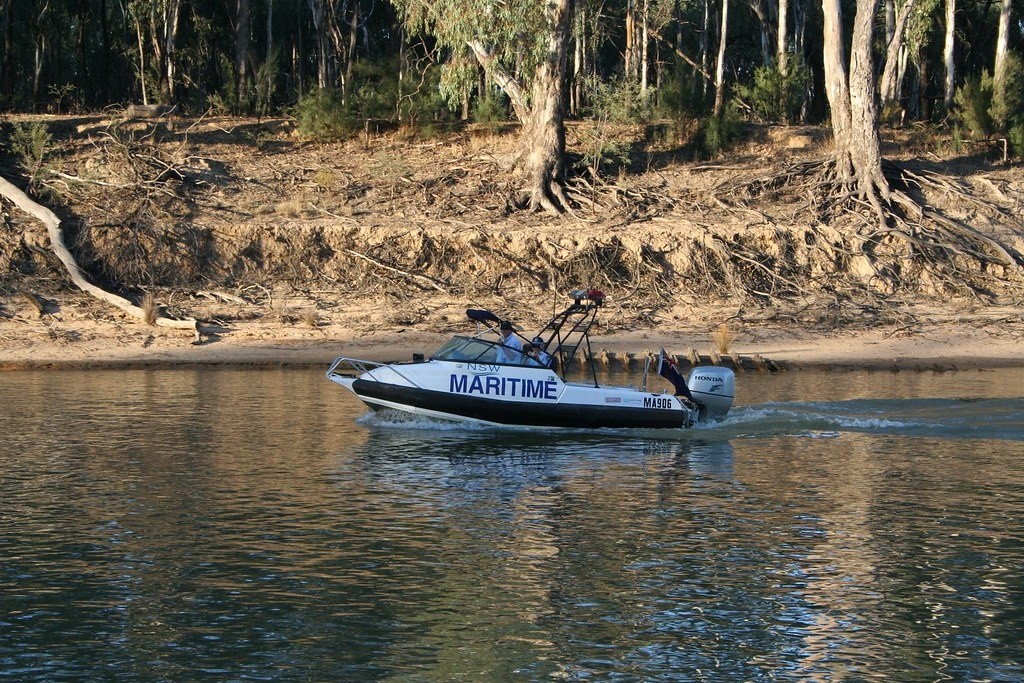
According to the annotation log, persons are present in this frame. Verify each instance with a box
[525,337,552,369]
[495,321,521,364]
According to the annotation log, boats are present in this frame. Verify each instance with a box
[325,289,734,429]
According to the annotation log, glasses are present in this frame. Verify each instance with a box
[500,327,508,330]
[532,345,540,348]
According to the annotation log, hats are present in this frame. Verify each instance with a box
[531,336,543,344]
[501,320,511,327]
